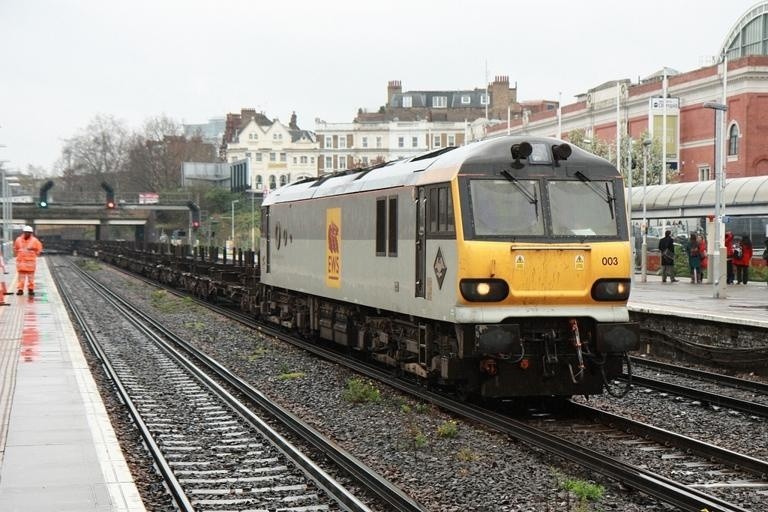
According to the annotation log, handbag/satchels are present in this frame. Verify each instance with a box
[664,248,677,261]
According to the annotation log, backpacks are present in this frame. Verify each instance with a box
[689,247,698,257]
[734,242,744,260]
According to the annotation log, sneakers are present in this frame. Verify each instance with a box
[15,288,36,296]
[689,277,703,284]
[727,279,748,285]
[670,279,680,281]
[662,279,666,282]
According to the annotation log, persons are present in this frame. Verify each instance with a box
[732,233,753,285]
[13,224,43,297]
[0,242,7,305]
[696,234,708,282]
[686,233,701,285]
[725,231,735,285]
[659,230,680,282]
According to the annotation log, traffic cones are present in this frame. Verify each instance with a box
[0,279,14,306]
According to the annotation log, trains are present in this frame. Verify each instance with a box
[42,134,640,404]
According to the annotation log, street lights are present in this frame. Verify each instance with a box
[641,139,653,282]
[703,102,728,299]
[251,167,263,253]
[232,199,240,241]
[0,144,22,263]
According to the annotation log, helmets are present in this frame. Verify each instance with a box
[22,225,34,233]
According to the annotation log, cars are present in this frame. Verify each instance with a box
[646,225,690,251]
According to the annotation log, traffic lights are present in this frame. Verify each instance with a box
[186,199,200,230]
[39,181,54,208]
[100,180,115,209]
